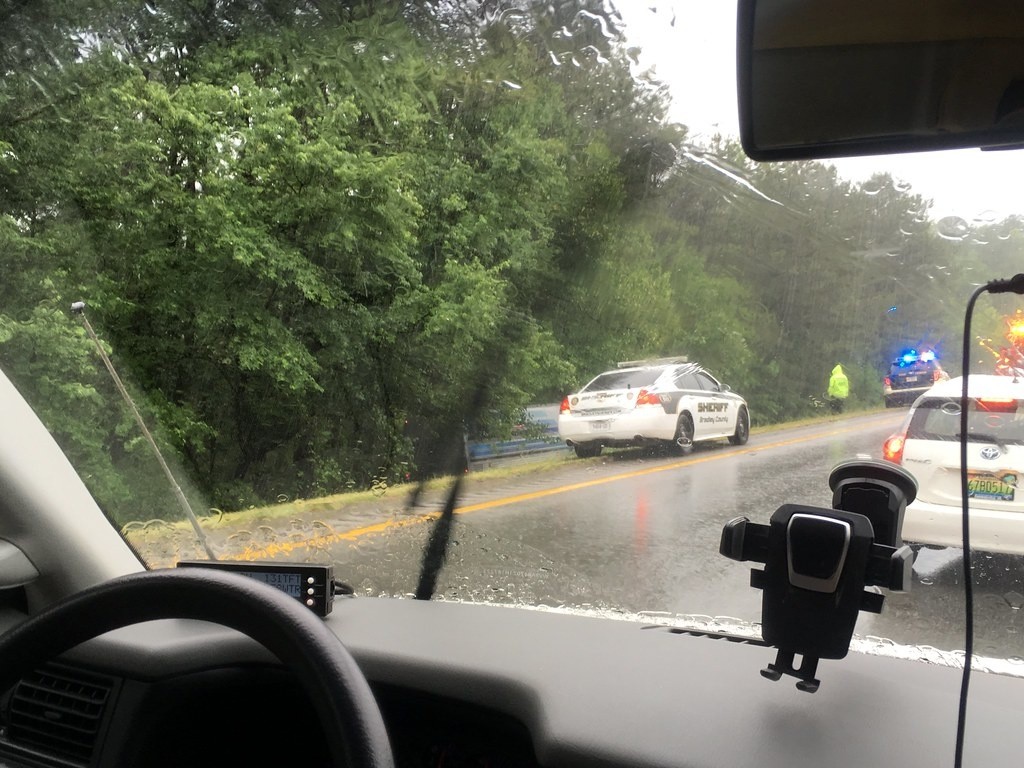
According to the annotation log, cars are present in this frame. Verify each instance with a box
[557,355,753,451]
[881,373,1024,563]
[883,354,949,406]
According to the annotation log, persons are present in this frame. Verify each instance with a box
[827,364,848,416]
